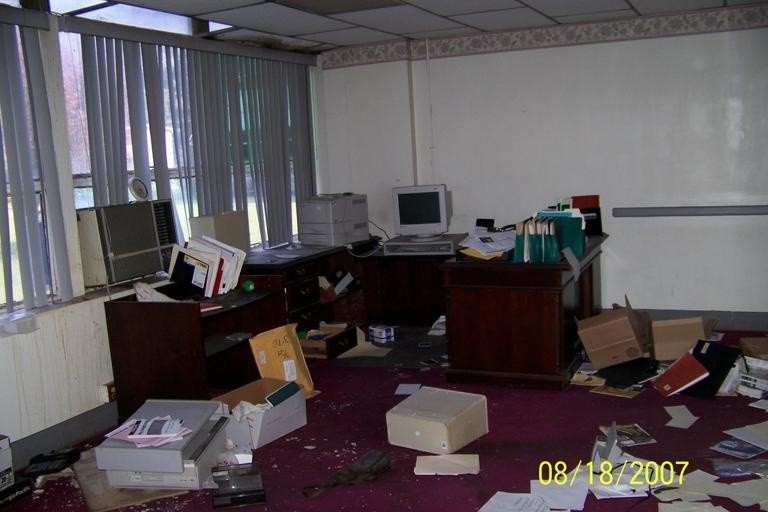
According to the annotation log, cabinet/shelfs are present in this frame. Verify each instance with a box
[100,269,285,434]
[354,244,470,327]
[429,228,611,391]
[235,228,381,365]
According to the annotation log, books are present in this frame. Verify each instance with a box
[168,234,246,299]
[653,351,712,397]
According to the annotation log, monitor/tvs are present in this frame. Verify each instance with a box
[391,184,453,243]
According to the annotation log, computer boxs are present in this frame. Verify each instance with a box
[383,233,468,256]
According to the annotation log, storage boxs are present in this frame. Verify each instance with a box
[651,316,706,361]
[384,385,491,453]
[211,375,307,456]
[738,338,767,361]
[0,435,36,511]
[572,293,649,373]
[189,210,249,261]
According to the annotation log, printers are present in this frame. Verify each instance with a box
[298,195,369,247]
